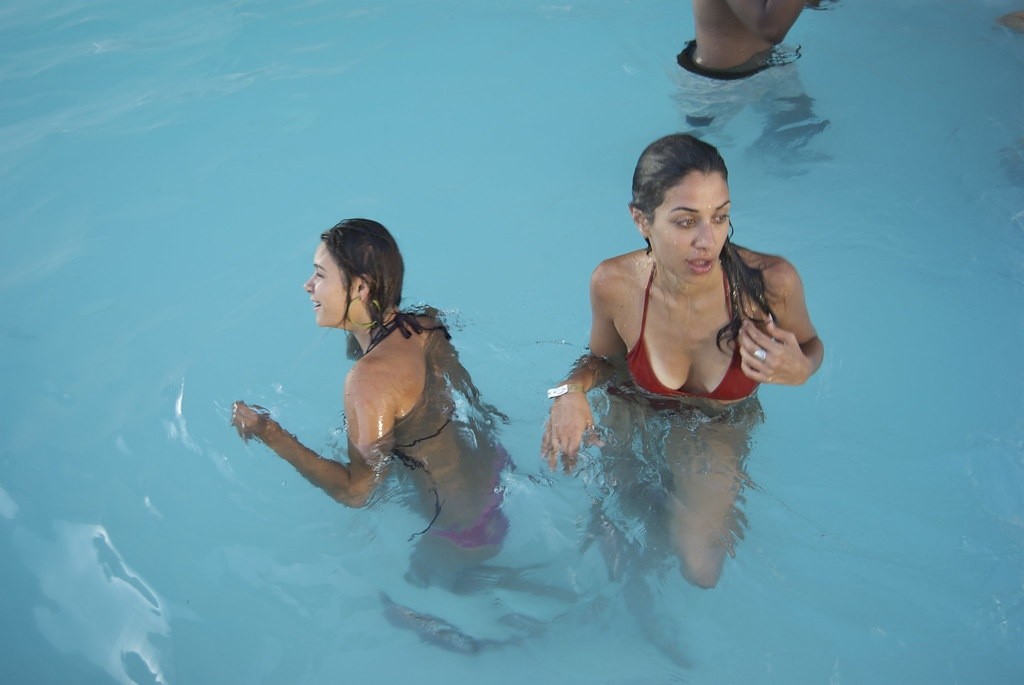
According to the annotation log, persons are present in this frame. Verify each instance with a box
[233,217,515,596]
[543,132,823,591]
[675,0,830,153]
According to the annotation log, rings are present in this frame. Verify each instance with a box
[753,349,768,363]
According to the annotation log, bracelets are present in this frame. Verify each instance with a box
[548,383,588,398]
